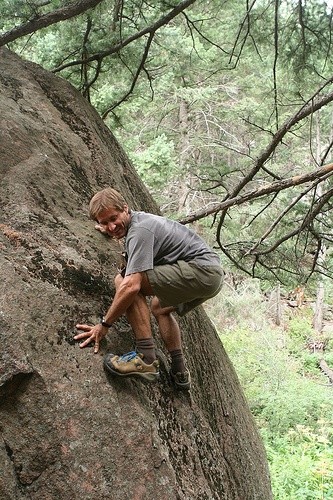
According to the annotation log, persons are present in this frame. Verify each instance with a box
[73,187,224,391]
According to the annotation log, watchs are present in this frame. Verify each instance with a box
[101,317,113,328]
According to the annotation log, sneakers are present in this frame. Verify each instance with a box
[168,365,192,392]
[103,351,161,384]
